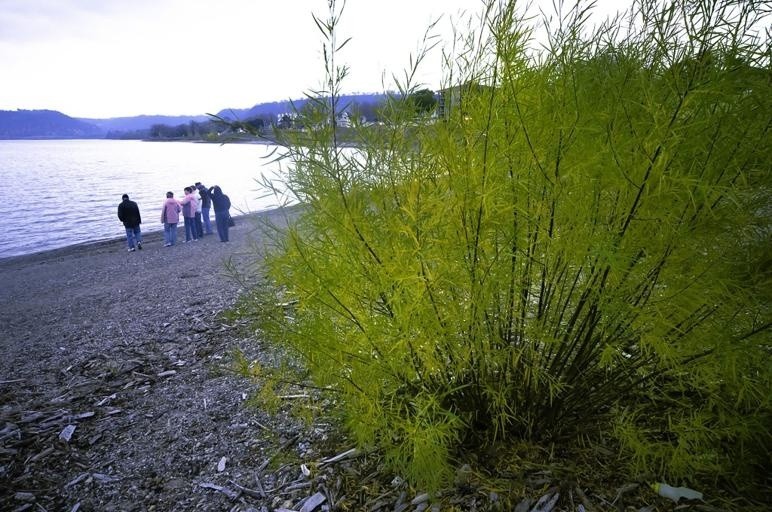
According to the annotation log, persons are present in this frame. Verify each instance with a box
[195,182,213,234]
[207,185,231,242]
[161,191,181,247]
[178,187,198,243]
[118,194,142,251]
[189,186,203,239]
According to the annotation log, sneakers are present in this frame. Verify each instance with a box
[164,243,172,247]
[127,248,135,252]
[182,232,213,243]
[137,241,142,250]
[220,240,229,243]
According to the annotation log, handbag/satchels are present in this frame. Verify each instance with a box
[228,214,235,227]
